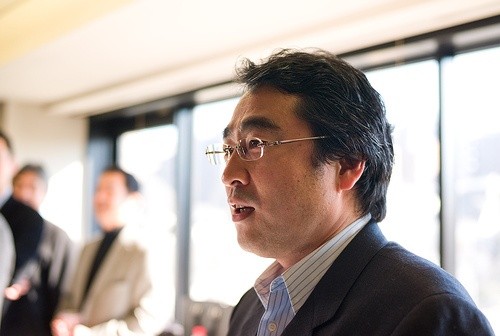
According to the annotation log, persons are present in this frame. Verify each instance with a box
[49,167,175,336]
[205,48,495,336]
[0,133,78,336]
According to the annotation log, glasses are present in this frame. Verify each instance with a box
[207,136,330,166]
